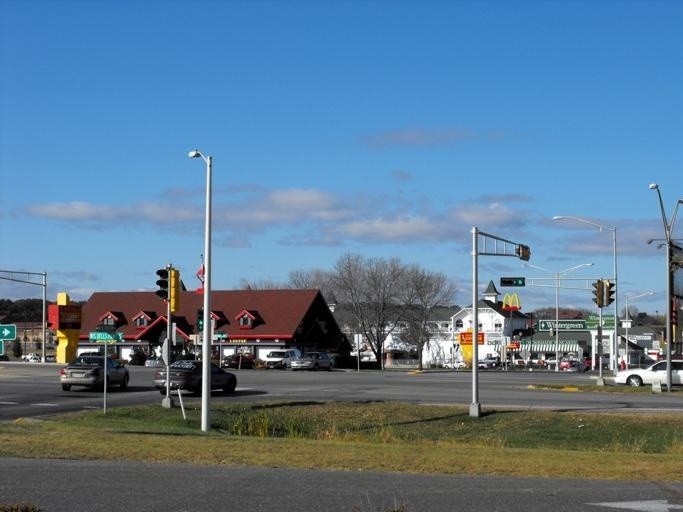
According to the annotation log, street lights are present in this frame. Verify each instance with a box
[649,183,683,392]
[625,290,656,370]
[520,263,595,372]
[552,215,618,376]
[187,151,212,432]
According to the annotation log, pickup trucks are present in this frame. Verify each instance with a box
[20,353,40,363]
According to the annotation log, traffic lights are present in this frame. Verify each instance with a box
[155,269,171,302]
[606,279,615,306]
[592,279,604,308]
[499,276,525,287]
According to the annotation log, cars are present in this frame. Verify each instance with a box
[544,357,560,367]
[478,357,500,369]
[221,353,254,369]
[59,355,130,391]
[430,359,465,369]
[152,360,237,395]
[560,360,583,371]
[264,349,335,371]
[614,359,683,387]
